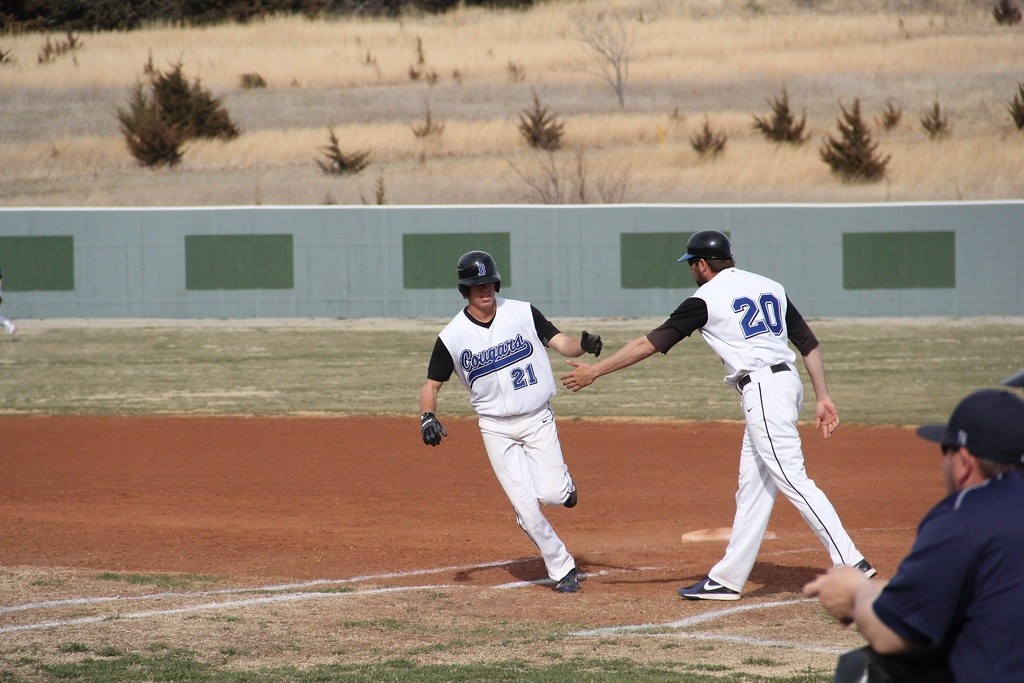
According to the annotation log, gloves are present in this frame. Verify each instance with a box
[421,412,448,446]
[580,331,602,357]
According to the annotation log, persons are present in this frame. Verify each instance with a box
[560,230,878,600]
[802,389,1024,683]
[419,251,603,593]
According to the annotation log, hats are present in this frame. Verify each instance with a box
[916,389,1024,460]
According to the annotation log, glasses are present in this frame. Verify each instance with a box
[940,444,955,455]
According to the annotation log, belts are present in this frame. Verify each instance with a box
[738,363,790,390]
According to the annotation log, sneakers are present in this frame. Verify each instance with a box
[854,560,878,579]
[555,565,579,592]
[678,576,742,600]
[564,479,577,508]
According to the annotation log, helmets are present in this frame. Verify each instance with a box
[677,230,731,262]
[457,251,502,299]
